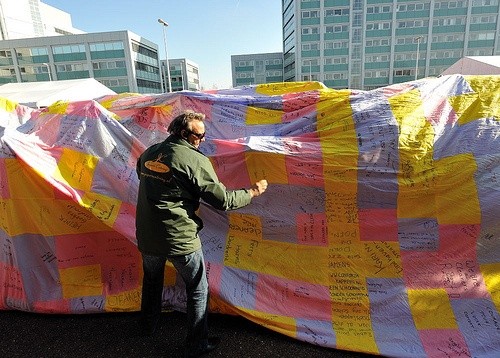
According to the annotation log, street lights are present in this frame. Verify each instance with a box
[157,18,172,91]
[415,36,423,80]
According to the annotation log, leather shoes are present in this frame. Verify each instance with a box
[189,336,221,357]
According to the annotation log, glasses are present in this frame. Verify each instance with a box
[185,128,205,139]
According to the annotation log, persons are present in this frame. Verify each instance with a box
[135,112,268,353]
